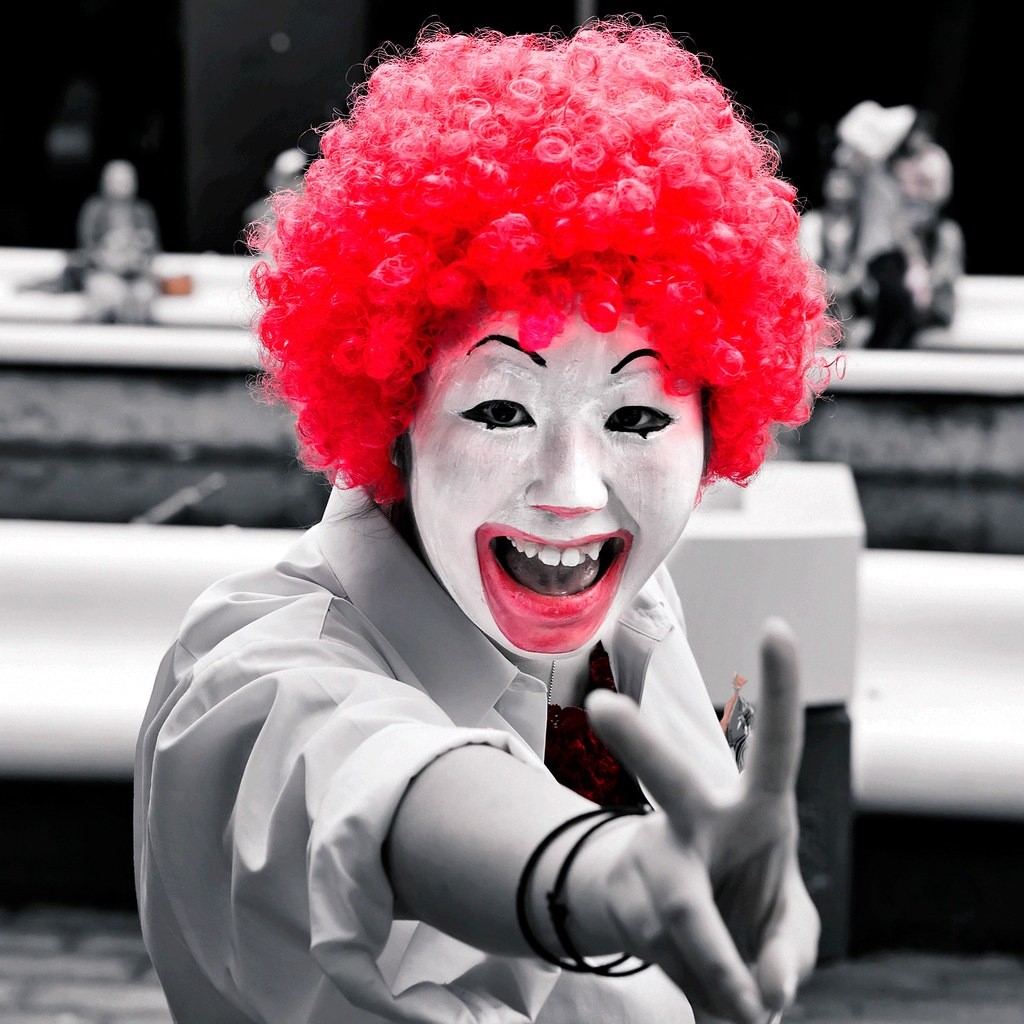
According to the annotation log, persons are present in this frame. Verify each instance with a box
[132,16,835,1024]
[76,101,963,355]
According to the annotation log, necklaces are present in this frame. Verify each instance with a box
[545,661,557,701]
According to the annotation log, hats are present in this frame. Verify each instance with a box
[834,101,917,164]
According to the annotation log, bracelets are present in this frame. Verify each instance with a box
[512,802,658,977]
